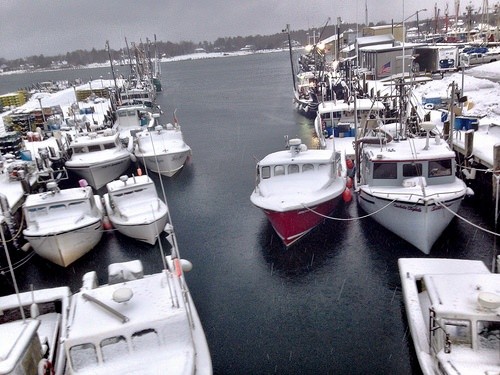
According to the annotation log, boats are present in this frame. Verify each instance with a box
[21,167,106,268]
[112,104,160,145]
[65,253,212,375]
[134,110,190,178]
[398,257,500,375]
[352,78,467,254]
[298,44,326,70]
[249,138,348,248]
[102,174,168,245]
[64,128,134,191]
[0,285,71,375]
[294,71,352,115]
[313,98,386,160]
